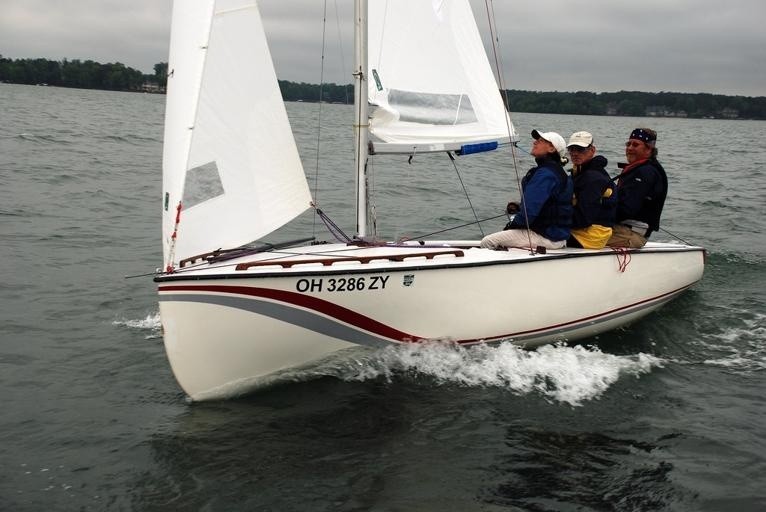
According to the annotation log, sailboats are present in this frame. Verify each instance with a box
[151,1,710,412]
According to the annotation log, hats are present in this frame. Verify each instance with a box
[531,129,567,158]
[629,127,657,143]
[565,131,596,150]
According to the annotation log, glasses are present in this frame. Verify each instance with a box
[625,141,645,147]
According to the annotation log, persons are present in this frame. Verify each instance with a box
[480,128,668,249]
